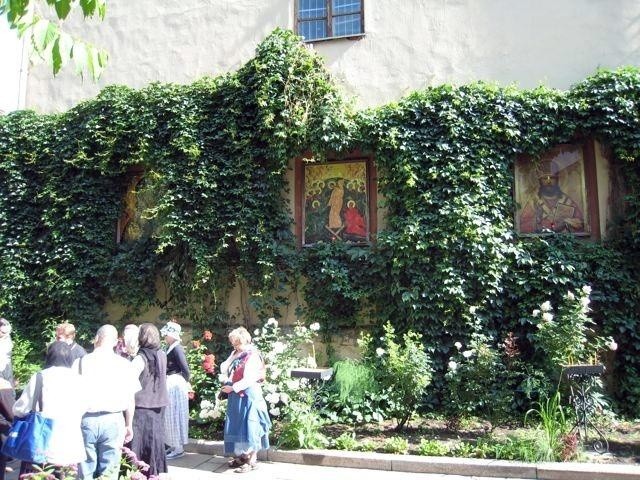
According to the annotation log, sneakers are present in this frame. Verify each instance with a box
[166,449,184,459]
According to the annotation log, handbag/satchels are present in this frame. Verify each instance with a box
[218,391,228,400]
[2,411,55,465]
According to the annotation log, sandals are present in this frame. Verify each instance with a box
[229,457,247,466]
[235,462,256,472]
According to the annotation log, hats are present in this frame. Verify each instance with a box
[160,322,181,340]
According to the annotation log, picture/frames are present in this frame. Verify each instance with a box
[113,168,152,245]
[511,131,603,250]
[293,152,378,253]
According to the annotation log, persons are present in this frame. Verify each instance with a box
[307,176,367,242]
[519,158,586,233]
[219,325,273,474]
[0,318,191,479]
